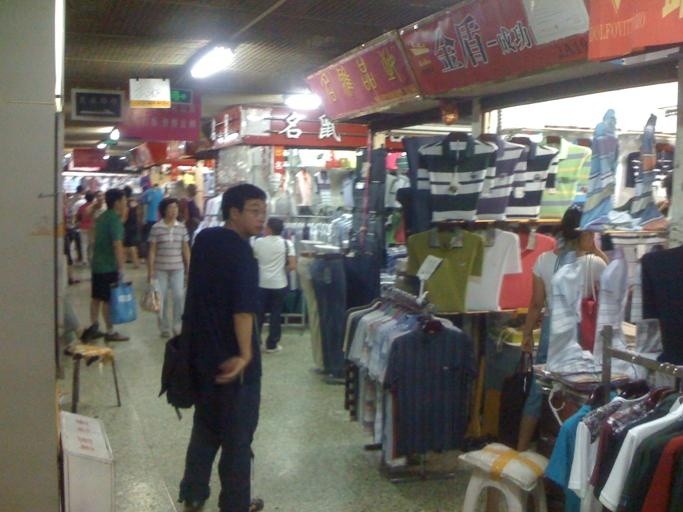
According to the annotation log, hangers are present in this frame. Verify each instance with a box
[586,354,683,405]
[381,286,442,333]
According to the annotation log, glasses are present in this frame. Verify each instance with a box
[236,206,270,216]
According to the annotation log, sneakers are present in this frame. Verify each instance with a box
[248,496,264,510]
[265,343,282,353]
[177,482,206,509]
[86,322,181,342]
[67,258,87,285]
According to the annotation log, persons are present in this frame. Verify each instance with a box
[253,216,297,352]
[177,183,265,511]
[63,175,202,343]
[515,203,610,452]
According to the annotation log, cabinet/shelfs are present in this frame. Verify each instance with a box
[441,128,675,225]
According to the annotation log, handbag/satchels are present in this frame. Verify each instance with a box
[109,285,138,324]
[580,253,598,353]
[140,287,162,313]
[497,350,546,452]
[158,334,201,419]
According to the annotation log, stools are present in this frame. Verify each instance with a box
[63,344,120,413]
[464,468,547,512]
